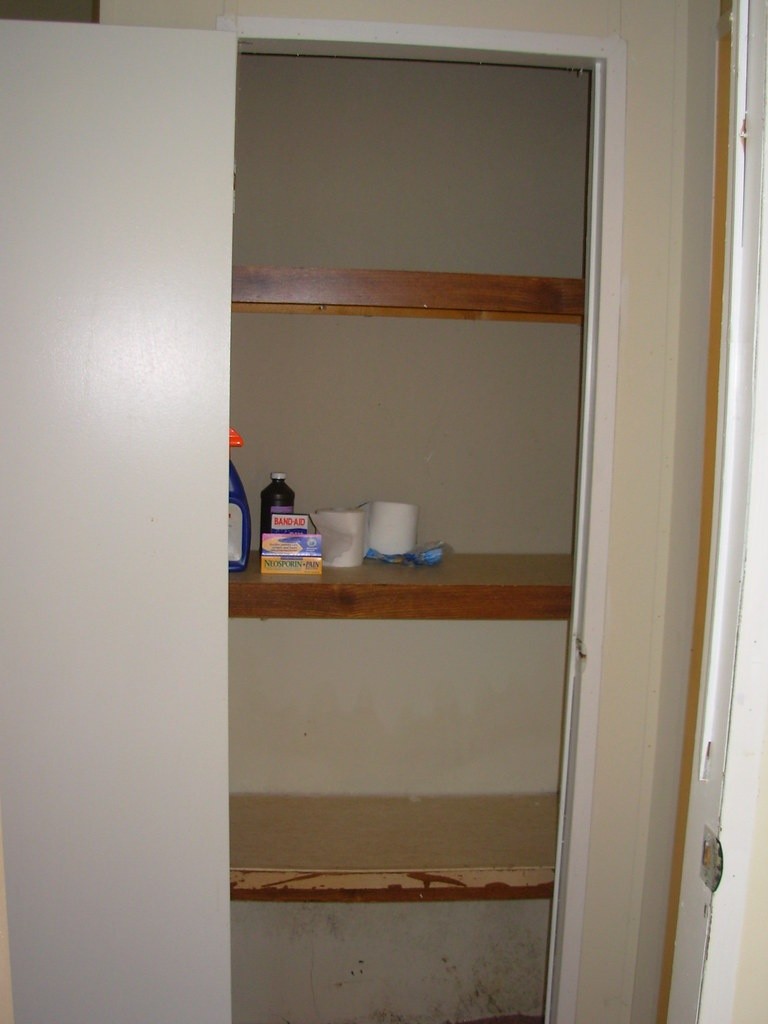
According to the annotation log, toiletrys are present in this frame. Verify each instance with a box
[364,547,443,566]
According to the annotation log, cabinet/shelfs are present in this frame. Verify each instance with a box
[228,266,593,902]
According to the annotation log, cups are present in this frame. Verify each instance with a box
[311,509,364,566]
[370,501,418,556]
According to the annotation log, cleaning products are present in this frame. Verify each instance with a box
[228,428,252,573]
[260,471,296,558]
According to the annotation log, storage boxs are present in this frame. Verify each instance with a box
[262,533,323,557]
[270,513,309,534]
[261,554,323,575]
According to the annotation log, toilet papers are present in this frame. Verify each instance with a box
[309,508,367,567]
[362,498,419,556]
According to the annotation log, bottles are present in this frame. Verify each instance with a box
[259,472,294,555]
